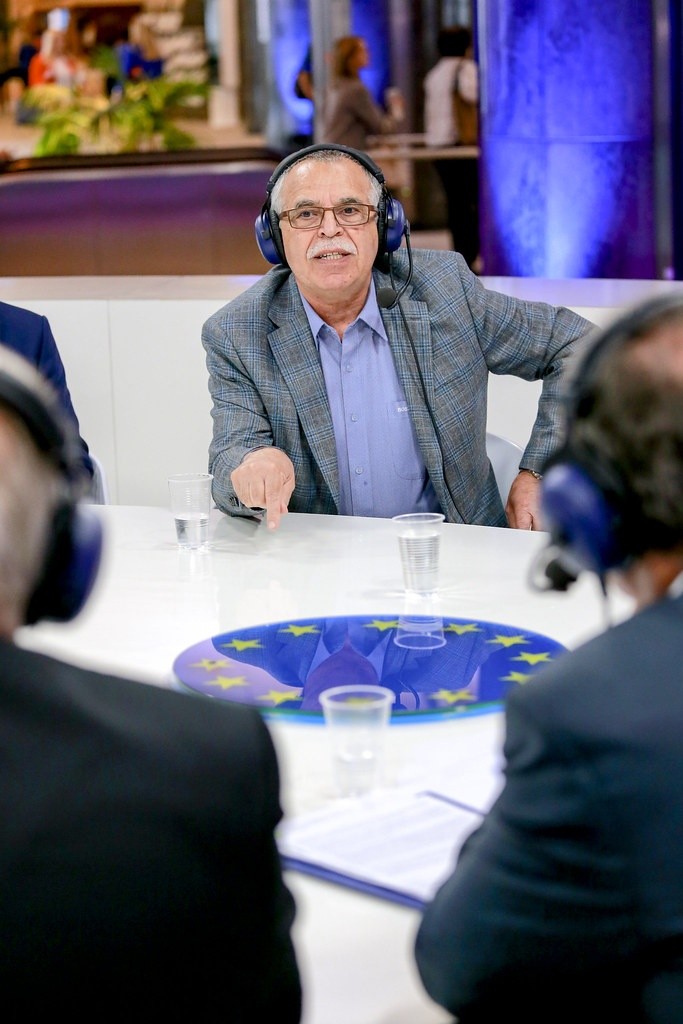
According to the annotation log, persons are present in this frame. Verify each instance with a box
[0,345,305,1024]
[16,9,152,123]
[202,144,607,536]
[0,302,95,503]
[424,26,481,274]
[414,295,683,1024]
[319,36,401,152]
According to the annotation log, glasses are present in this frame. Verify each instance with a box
[276,202,380,229]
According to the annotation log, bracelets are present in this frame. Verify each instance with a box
[531,470,544,480]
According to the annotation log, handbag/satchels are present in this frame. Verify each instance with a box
[451,59,479,143]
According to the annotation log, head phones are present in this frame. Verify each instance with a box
[253,143,410,264]
[541,288,683,572]
[0,344,106,625]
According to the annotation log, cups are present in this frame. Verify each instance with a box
[318,685,396,770]
[394,595,448,649]
[169,473,213,546]
[392,513,445,593]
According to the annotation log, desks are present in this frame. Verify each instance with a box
[12,505,636,1024]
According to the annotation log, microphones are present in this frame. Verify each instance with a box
[528,541,578,593]
[376,236,414,309]
